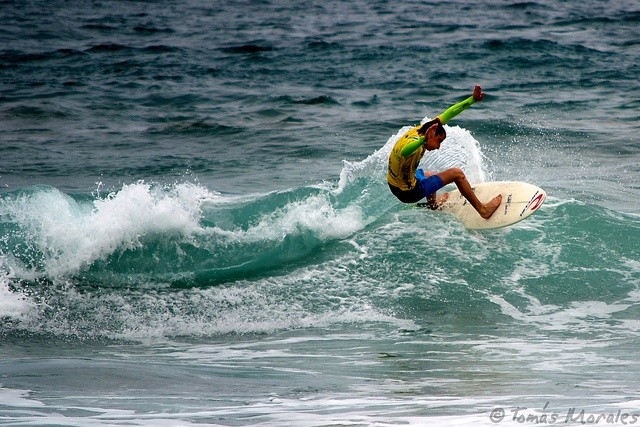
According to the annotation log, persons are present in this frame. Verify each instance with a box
[386,85,502,221]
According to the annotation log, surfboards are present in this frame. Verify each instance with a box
[434,181,547,230]
